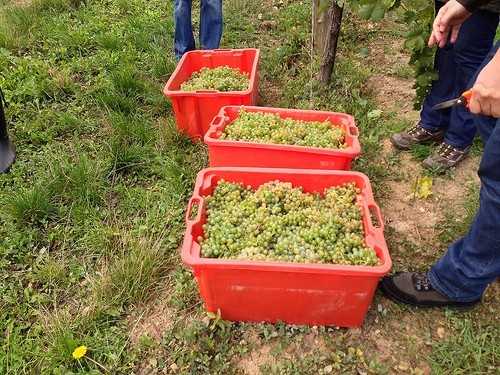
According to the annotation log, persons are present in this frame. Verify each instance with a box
[391,0,499,176]
[172,1,224,62]
[382,34,499,312]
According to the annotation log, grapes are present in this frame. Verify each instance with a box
[181,64,251,91]
[218,104,349,149]
[197,178,381,266]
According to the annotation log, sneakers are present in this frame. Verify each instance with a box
[380,272,482,312]
[390,125,447,150]
[421,142,471,172]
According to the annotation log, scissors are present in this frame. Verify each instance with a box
[433,88,473,114]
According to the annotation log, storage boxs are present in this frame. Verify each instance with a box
[181,167,395,328]
[204,106,361,170]
[163,48,260,141]
